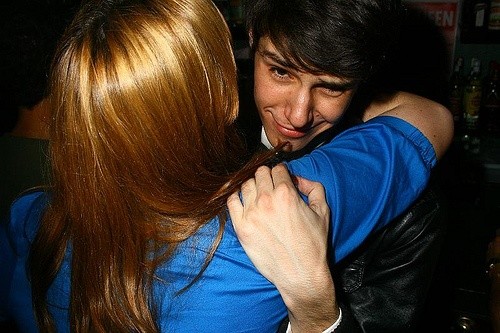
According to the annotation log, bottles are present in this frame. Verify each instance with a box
[445,56,499,126]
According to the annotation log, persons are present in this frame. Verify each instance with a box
[196,0,480,333]
[0,33,59,214]
[1,0,456,332]
